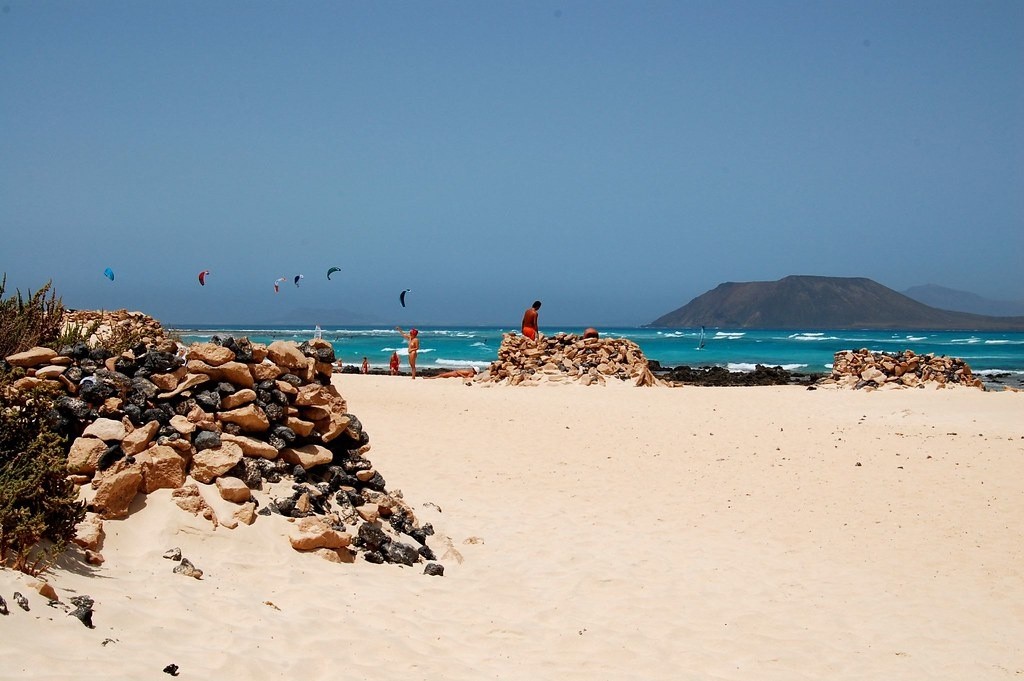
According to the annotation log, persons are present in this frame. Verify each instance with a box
[390,351,401,376]
[584,328,599,339]
[397,327,419,379]
[421,366,480,380]
[335,358,342,373]
[362,356,370,374]
[521,300,541,341]
